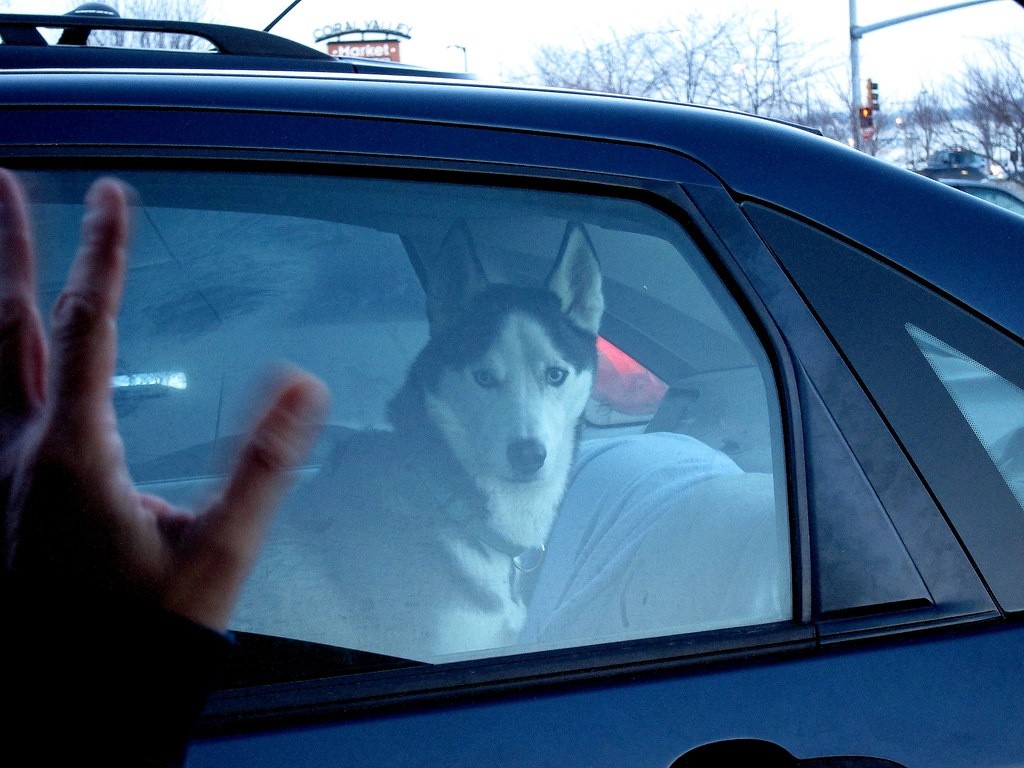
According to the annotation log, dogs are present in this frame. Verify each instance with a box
[231,218,607,665]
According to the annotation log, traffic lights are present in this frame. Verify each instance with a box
[866,79,881,114]
[859,106,873,129]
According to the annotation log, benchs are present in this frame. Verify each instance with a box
[524,431,791,644]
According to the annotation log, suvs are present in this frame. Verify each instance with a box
[1,10,1024,765]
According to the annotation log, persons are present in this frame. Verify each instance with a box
[0,162,334,768]
